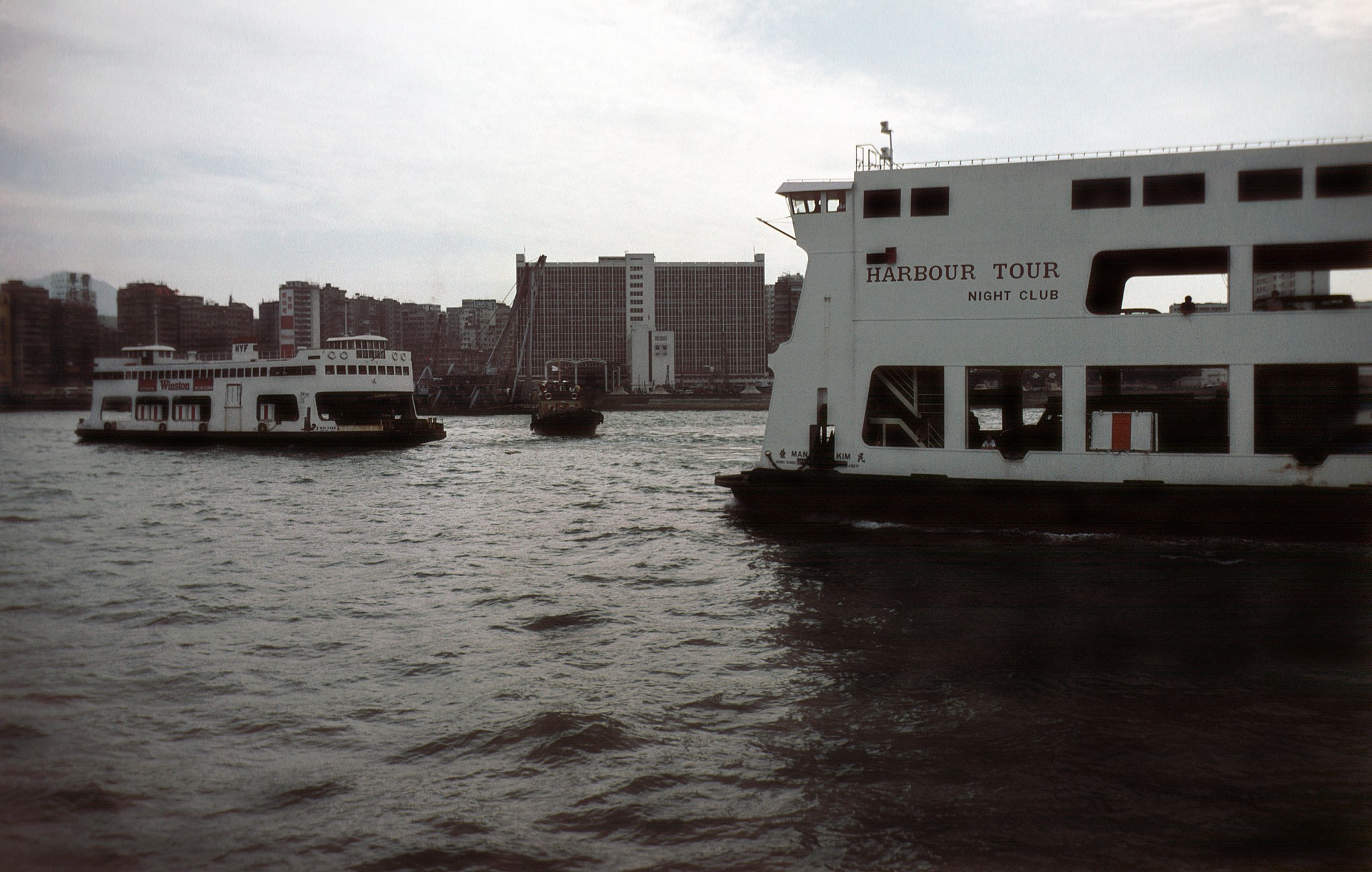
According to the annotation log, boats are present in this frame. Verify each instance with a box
[73,299,446,453]
[413,254,548,418]
[714,120,1372,549]
[530,377,603,439]
